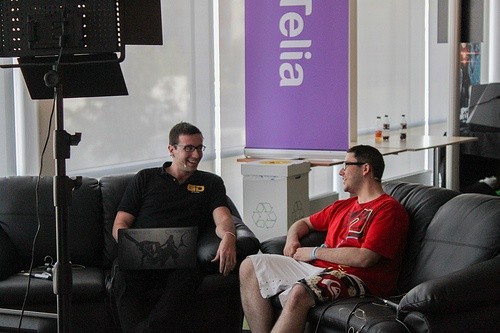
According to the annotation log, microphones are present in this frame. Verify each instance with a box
[382,298,399,311]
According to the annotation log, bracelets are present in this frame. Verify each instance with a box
[222,231,237,239]
[311,246,319,260]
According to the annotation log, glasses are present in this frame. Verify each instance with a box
[343,161,365,171]
[173,145,206,152]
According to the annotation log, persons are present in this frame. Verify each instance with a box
[107,121,240,333]
[239,142,408,333]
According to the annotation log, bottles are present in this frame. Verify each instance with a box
[383,115,390,142]
[399,115,407,141]
[375,116,382,143]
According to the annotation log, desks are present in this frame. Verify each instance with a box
[376,134,479,189]
[240,158,311,254]
[296,147,408,167]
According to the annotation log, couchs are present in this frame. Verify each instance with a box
[261,179,500,333]
[0,170,260,333]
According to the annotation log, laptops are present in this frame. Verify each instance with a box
[119,227,199,272]
